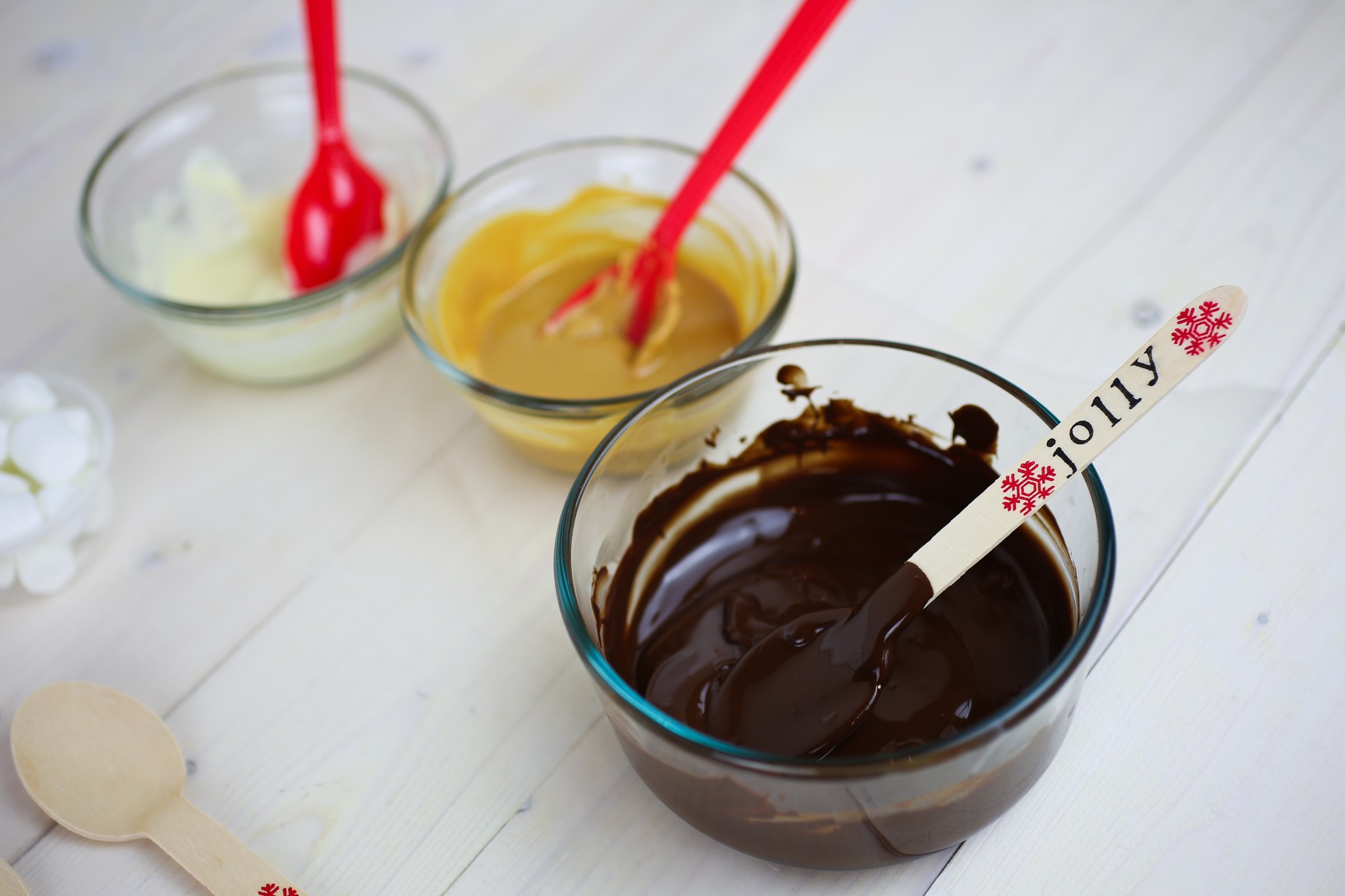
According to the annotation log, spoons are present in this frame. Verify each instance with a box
[10,681,307,896]
[544,1,851,343]
[712,285,1247,760]
[284,0,391,298]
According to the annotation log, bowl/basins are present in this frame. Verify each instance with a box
[552,337,1118,871]
[398,136,799,476]
[76,61,457,388]
[0,373,111,598]
